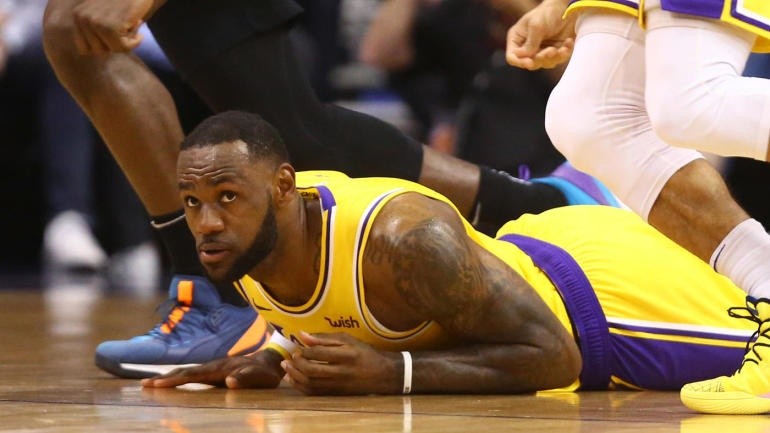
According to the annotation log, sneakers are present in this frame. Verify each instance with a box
[519,161,628,209]
[95,274,259,379]
[679,295,770,414]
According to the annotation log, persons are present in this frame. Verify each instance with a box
[0,0,770,416]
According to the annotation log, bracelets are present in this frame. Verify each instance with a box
[401,352,413,396]
